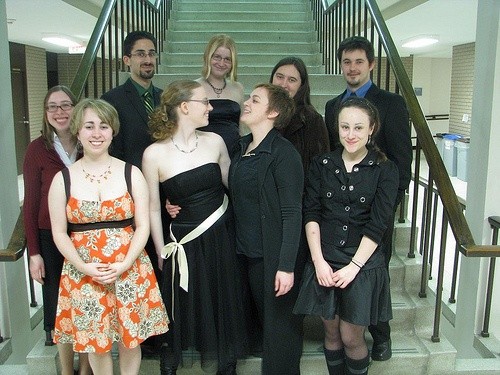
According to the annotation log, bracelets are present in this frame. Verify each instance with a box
[349,256,364,270]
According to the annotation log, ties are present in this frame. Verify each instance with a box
[348,92,357,97]
[143,92,155,120]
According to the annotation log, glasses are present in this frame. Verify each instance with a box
[213,54,232,63]
[187,98,210,105]
[127,52,156,58]
[45,104,73,111]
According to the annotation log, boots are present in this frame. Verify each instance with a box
[215,352,239,375]
[156,345,180,375]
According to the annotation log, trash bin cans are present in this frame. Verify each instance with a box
[455,137,470,182]
[443,135,463,177]
[434,132,444,161]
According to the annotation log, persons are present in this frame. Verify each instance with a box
[302,97,399,375]
[48,97,171,375]
[141,80,250,375]
[324,36,412,362]
[99,31,164,171]
[269,56,331,186]
[193,32,245,160]
[164,83,307,375]
[24,85,84,374]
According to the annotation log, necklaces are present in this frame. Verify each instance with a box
[81,157,114,184]
[171,129,199,154]
[204,77,226,99]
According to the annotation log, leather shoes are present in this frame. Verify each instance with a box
[370,328,392,361]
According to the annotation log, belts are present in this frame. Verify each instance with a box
[65,218,134,233]
